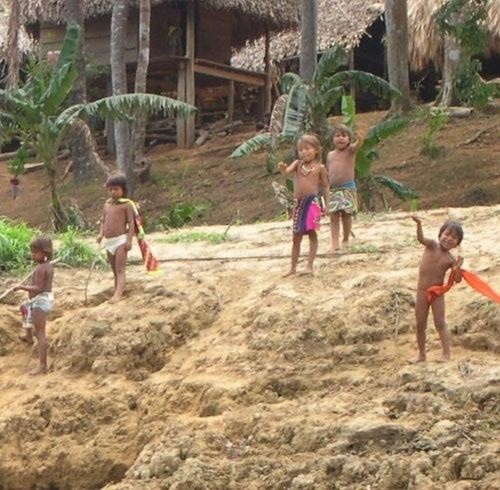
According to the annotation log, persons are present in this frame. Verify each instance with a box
[406,214,464,365]
[96,172,136,304]
[14,234,54,376]
[277,123,368,279]
